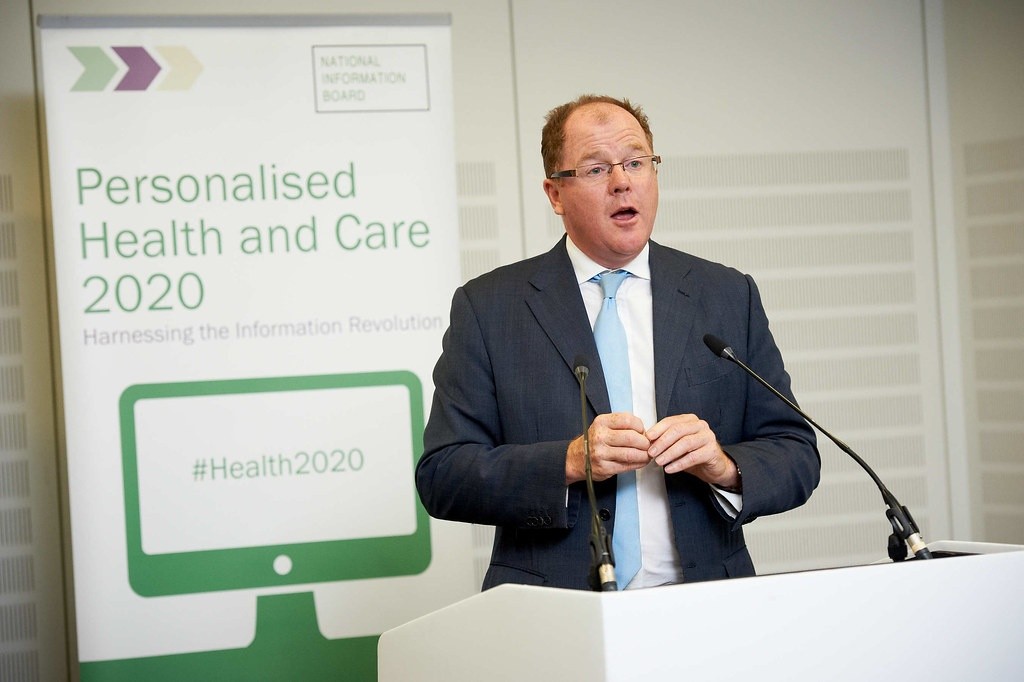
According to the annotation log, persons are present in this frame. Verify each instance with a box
[415,94,822,592]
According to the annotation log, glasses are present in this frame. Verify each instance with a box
[550,155,661,185]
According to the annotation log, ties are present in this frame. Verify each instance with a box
[587,268,644,591]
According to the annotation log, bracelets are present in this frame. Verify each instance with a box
[712,464,742,493]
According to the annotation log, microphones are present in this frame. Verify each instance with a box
[703,332,934,560]
[573,356,617,592]
